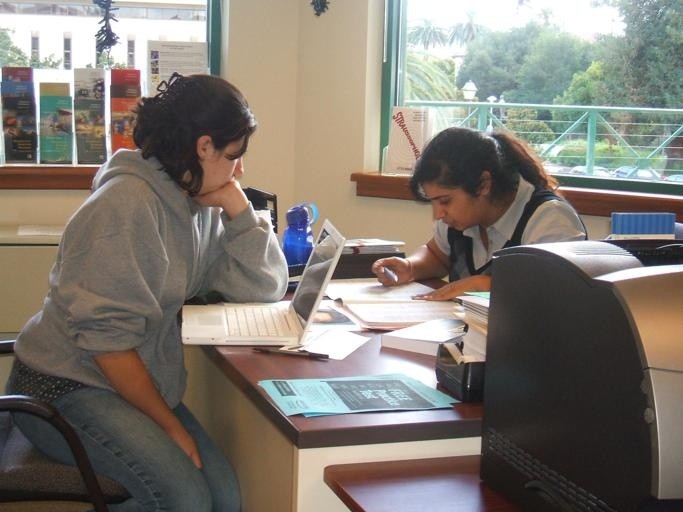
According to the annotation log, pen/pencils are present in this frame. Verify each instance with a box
[254,347,328,361]
[384,267,399,283]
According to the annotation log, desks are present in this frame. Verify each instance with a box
[323,453,548,512]
[173,273,483,512]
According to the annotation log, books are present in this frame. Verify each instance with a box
[381,316,466,356]
[1,66,141,165]
[338,239,405,257]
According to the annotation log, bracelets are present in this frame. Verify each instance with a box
[399,255,413,285]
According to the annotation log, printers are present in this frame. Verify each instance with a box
[480,237,683,512]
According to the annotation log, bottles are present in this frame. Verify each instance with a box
[281,201,319,292]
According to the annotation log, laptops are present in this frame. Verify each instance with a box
[180,218,345,346]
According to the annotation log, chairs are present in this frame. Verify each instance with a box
[0,340,130,512]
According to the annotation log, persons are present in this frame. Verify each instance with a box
[0,72,291,512]
[370,124,587,305]
